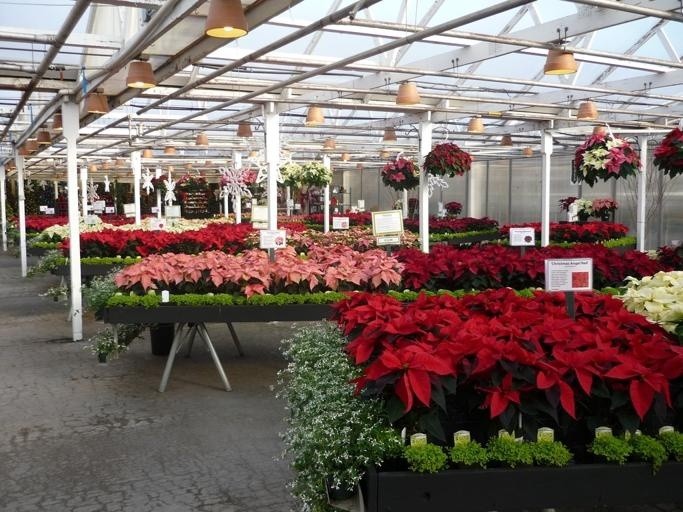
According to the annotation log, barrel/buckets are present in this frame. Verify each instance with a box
[150,323,175,356]
[174,323,198,358]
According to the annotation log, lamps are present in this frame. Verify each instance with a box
[125,53,157,89]
[397,82,422,107]
[304,105,326,125]
[8,113,603,177]
[205,2,248,38]
[83,87,111,113]
[543,29,578,75]
[577,100,599,120]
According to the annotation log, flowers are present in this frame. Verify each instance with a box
[6,122,683,356]
[268,292,683,511]
